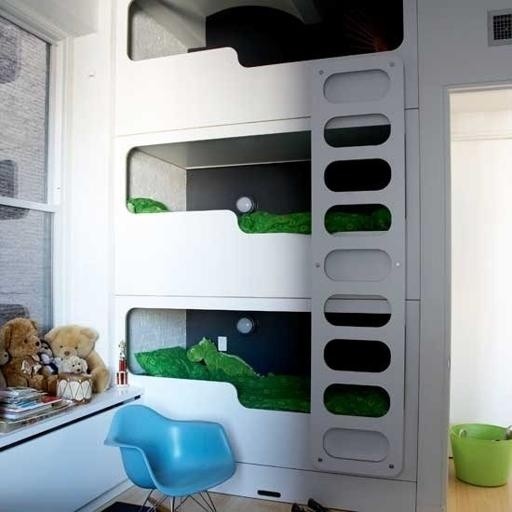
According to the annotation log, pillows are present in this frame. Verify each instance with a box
[130,197,171,213]
[133,346,204,378]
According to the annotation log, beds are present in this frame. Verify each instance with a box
[112,0,418,512]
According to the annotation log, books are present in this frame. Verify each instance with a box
[0,387,78,432]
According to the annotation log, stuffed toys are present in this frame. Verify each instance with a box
[59,356,88,374]
[1,317,47,390]
[187,337,268,381]
[0,351,9,389]
[44,324,112,393]
[38,339,59,375]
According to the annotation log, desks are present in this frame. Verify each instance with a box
[0,388,145,512]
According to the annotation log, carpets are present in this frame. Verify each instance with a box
[101,501,154,512]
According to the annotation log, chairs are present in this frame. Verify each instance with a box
[102,403,238,512]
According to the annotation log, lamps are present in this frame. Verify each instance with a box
[233,314,256,336]
[234,194,257,215]
